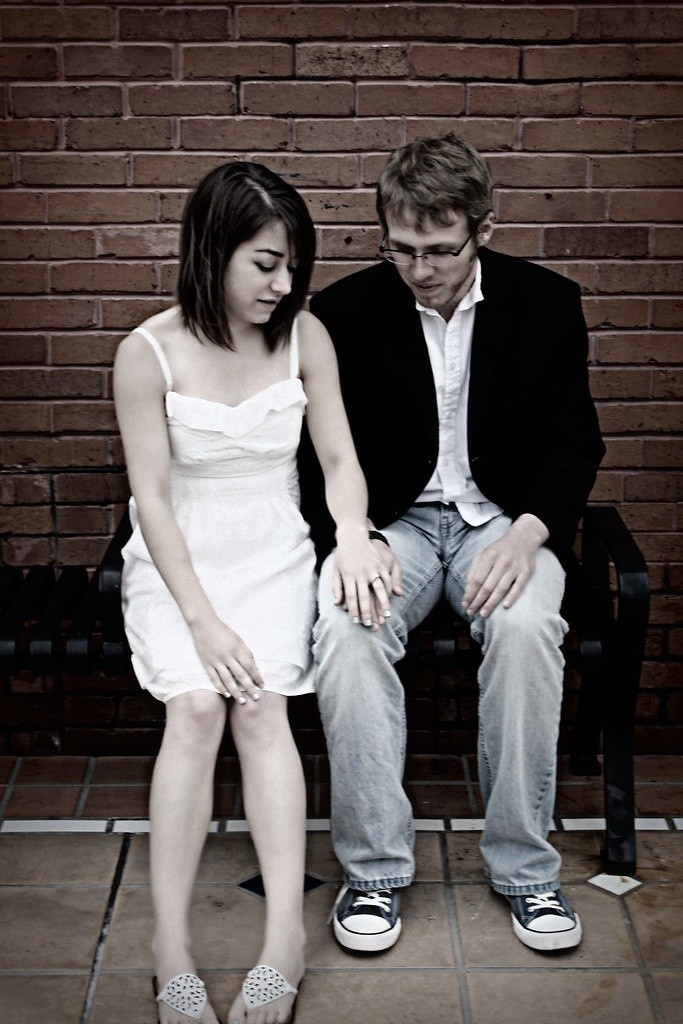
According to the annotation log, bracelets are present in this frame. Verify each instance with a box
[368,529,390,547]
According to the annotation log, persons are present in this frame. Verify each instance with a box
[295,130,609,953]
[105,157,395,1024]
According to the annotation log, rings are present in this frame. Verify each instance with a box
[368,573,380,584]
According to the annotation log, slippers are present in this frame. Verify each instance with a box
[152,973,224,1024]
[243,964,303,1024]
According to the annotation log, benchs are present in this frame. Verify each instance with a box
[87,497,649,888]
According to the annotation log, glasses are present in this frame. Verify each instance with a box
[379,207,494,266]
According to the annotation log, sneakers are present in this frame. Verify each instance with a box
[504,887,582,951]
[327,884,403,951]
